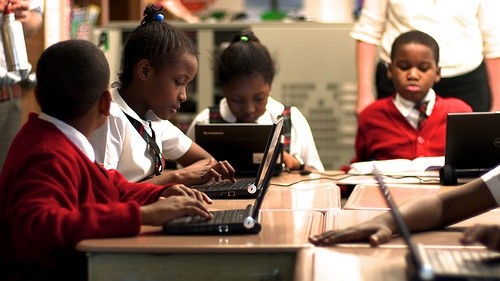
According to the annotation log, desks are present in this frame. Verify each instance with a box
[77,175,500,281]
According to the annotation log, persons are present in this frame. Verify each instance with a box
[337,29,474,200]
[348,0,500,111]
[176,28,326,176]
[308,165,500,253]
[87,4,238,187]
[0,38,215,281]
[0,0,51,93]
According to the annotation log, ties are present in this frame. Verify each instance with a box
[414,101,429,130]
[122,109,165,176]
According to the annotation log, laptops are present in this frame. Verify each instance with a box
[190,117,285,199]
[445,112,500,176]
[163,135,285,236]
[374,167,500,280]
[193,123,284,178]
